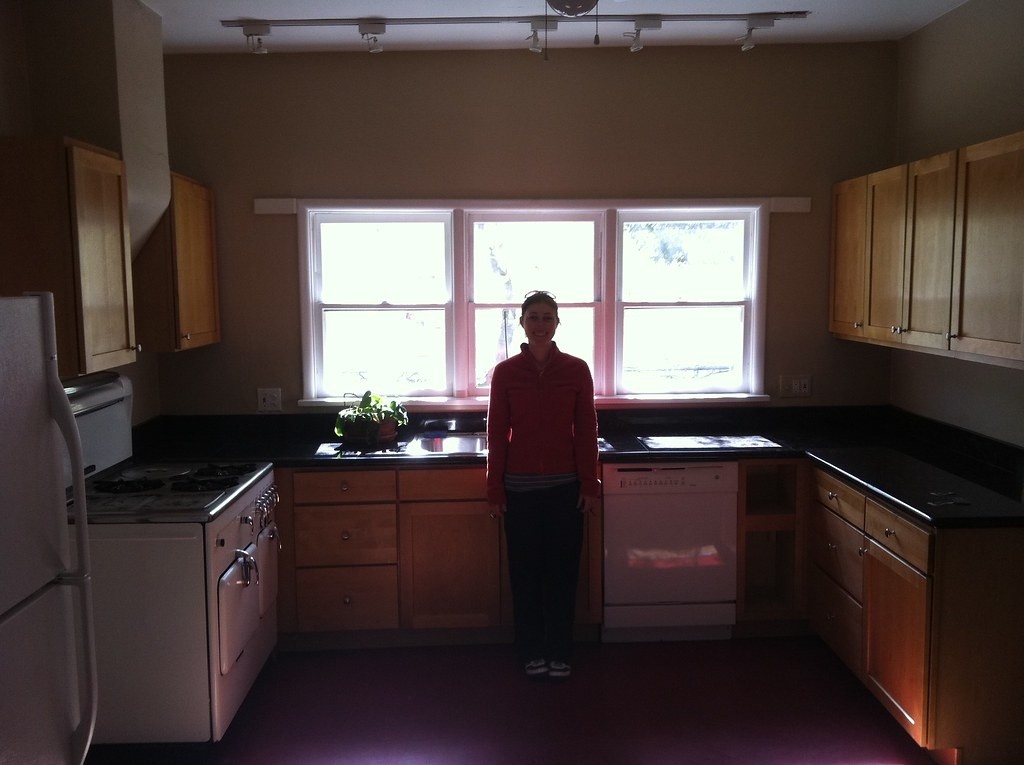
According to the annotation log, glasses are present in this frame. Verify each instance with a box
[524,290,556,299]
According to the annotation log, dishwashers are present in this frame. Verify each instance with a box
[602,462,739,646]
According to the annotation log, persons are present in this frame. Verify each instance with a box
[486,292,601,682]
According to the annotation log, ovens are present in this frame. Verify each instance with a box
[68,468,280,746]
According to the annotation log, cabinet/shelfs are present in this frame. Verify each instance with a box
[828,131,1024,371]
[738,459,809,639]
[274,464,603,647]
[809,466,1024,765]
[0,140,221,378]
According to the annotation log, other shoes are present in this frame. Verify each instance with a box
[548,660,571,676]
[522,654,549,676]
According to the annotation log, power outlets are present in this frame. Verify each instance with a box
[779,374,812,397]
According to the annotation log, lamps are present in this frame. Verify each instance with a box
[218,10,809,55]
[543,0,600,60]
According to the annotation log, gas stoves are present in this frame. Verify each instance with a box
[57,366,273,524]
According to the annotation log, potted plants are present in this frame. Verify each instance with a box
[334,389,408,451]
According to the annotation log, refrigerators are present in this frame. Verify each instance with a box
[1,289,98,765]
